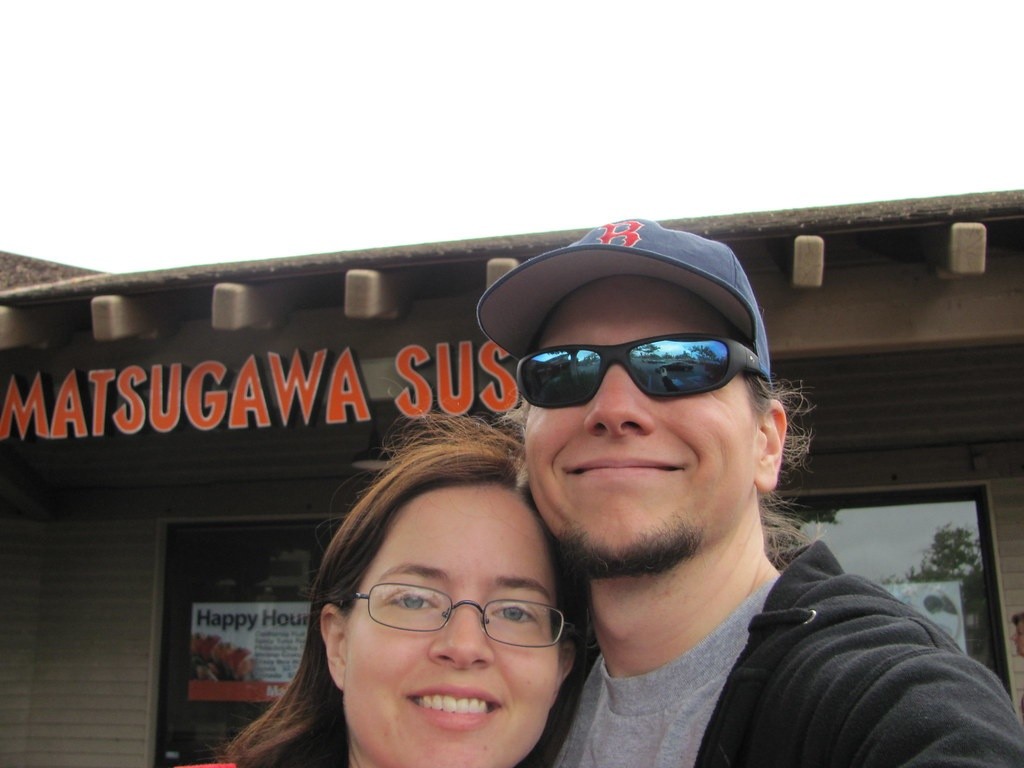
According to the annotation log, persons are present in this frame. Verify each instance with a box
[477,220,1024,768]
[213,416,590,768]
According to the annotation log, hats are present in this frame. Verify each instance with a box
[476,219,771,383]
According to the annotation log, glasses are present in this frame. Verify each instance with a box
[516,334,770,409]
[336,583,575,648]
